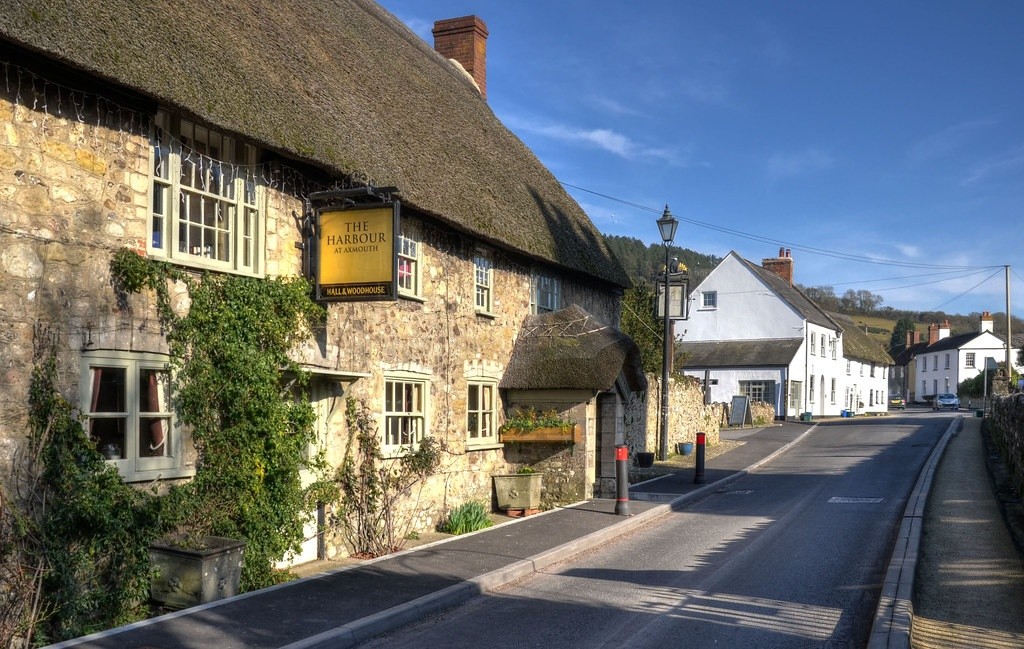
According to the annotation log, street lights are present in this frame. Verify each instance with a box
[657,205,679,461]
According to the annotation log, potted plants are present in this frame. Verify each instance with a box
[492,466,544,509]
[500,405,581,443]
[146,489,245,607]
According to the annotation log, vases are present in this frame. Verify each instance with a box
[679,442,693,455]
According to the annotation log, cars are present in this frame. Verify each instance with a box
[889,394,906,410]
[933,392,961,411]
